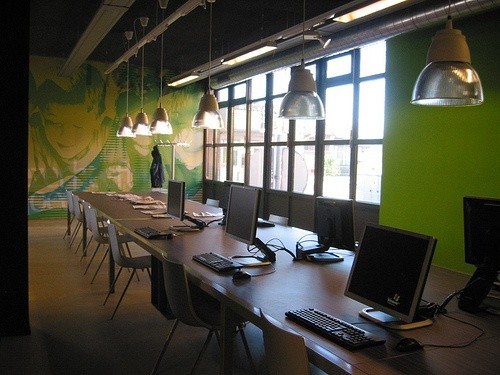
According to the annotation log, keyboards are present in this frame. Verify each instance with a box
[257,217,275,228]
[133,226,175,241]
[192,251,244,273]
[285,306,388,351]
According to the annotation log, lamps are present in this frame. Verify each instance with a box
[192,0,225,129]
[314,36,331,49]
[278,0,325,121]
[221,41,278,69]
[115,0,174,138]
[167,70,200,87]
[331,0,409,24]
[409,0,484,107]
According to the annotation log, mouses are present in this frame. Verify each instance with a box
[233,271,250,279]
[395,338,423,353]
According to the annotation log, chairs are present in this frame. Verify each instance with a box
[63,188,311,375]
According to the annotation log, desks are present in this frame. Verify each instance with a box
[111,216,500,375]
[72,191,223,294]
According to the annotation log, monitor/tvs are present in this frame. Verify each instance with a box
[343,221,438,330]
[220,180,275,277]
[294,197,355,263]
[166,180,204,232]
[457,195,500,315]
